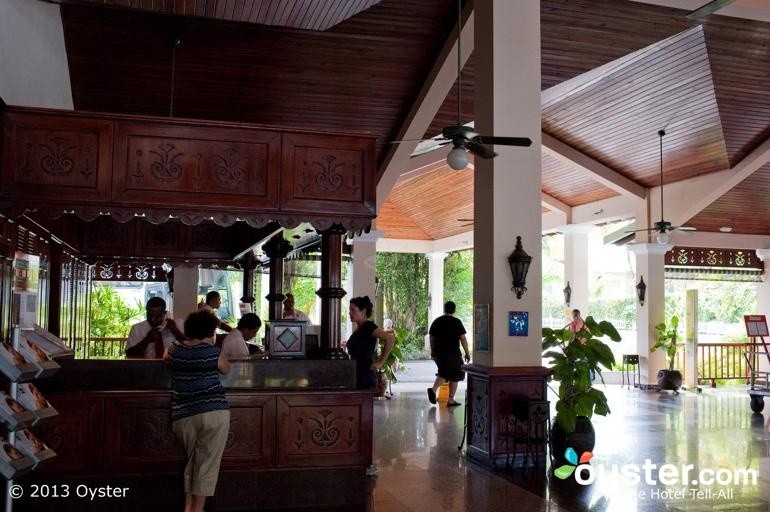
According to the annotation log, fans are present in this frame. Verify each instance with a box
[624,130,711,244]
[389,20,532,170]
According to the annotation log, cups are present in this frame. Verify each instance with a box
[259,345,265,359]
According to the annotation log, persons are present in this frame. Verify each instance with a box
[222,312,261,362]
[28,381,50,410]
[282,293,313,327]
[3,341,27,365]
[24,430,44,452]
[3,444,24,460]
[167,311,231,512]
[345,296,395,391]
[6,397,27,413]
[427,302,470,406]
[384,319,394,399]
[28,339,49,363]
[200,292,232,345]
[126,298,175,360]
[569,310,586,344]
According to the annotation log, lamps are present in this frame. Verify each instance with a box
[375,275,379,298]
[506,235,533,299]
[657,226,669,244]
[447,139,468,169]
[635,274,648,307]
[562,282,573,306]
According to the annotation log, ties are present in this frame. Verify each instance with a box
[154,332,164,359]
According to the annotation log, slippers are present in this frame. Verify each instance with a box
[427,388,436,404]
[447,401,462,406]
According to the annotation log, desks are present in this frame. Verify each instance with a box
[622,354,641,389]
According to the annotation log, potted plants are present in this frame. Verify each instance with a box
[650,316,684,389]
[373,328,407,400]
[544,315,622,466]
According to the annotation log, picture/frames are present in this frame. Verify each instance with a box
[472,304,490,353]
[508,311,531,337]
